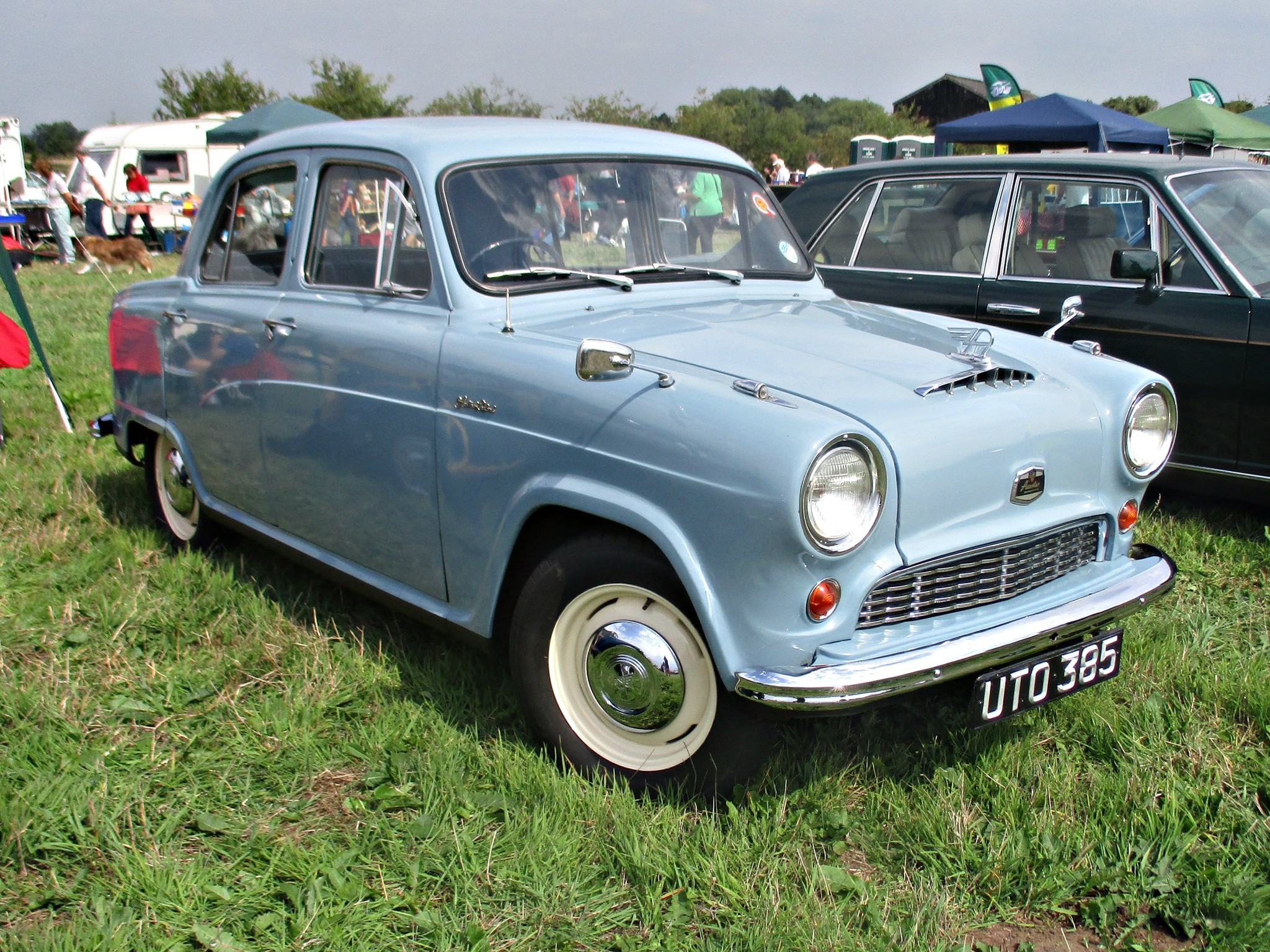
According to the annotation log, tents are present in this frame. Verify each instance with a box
[1137,96,1270,151]
[934,93,1169,155]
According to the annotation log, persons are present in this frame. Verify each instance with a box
[335,176,360,247]
[74,145,112,241]
[495,164,629,268]
[1056,183,1092,239]
[123,164,165,255]
[169,321,229,407]
[234,183,288,248]
[806,152,825,179]
[34,158,77,266]
[764,153,791,184]
[675,164,723,253]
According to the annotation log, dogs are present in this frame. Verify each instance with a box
[73,234,162,276]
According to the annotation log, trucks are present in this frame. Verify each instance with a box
[60,110,248,235]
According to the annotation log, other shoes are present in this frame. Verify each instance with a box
[599,235,615,248]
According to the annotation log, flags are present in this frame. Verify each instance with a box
[1189,78,1225,109]
[980,62,1025,155]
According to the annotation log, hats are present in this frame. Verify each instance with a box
[772,159,785,166]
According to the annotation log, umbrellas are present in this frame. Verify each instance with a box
[208,97,347,145]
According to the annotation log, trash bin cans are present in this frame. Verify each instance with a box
[163,234,186,253]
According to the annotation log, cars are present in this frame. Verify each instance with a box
[662,148,1269,505]
[84,116,1185,808]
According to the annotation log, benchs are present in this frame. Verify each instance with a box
[824,181,990,272]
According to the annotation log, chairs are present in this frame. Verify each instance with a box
[951,212,1054,279]
[1057,204,1146,283]
